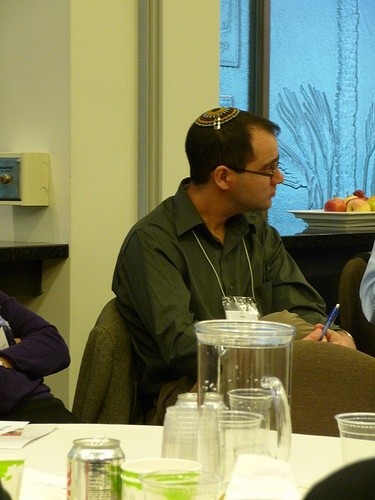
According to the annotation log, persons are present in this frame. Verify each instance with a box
[0,291,81,424]
[112,109,356,426]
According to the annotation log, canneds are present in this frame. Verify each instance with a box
[67,436,125,499]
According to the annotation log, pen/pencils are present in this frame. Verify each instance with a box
[319,303,341,342]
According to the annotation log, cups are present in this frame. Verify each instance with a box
[335,412,375,464]
[120,387,273,500]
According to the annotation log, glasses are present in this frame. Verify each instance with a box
[212,162,286,179]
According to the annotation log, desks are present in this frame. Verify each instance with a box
[1,418,375,500]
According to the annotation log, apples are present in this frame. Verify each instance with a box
[324,190,375,213]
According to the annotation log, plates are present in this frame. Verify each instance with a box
[286,209,375,228]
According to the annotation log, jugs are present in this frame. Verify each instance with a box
[194,319,295,491]
